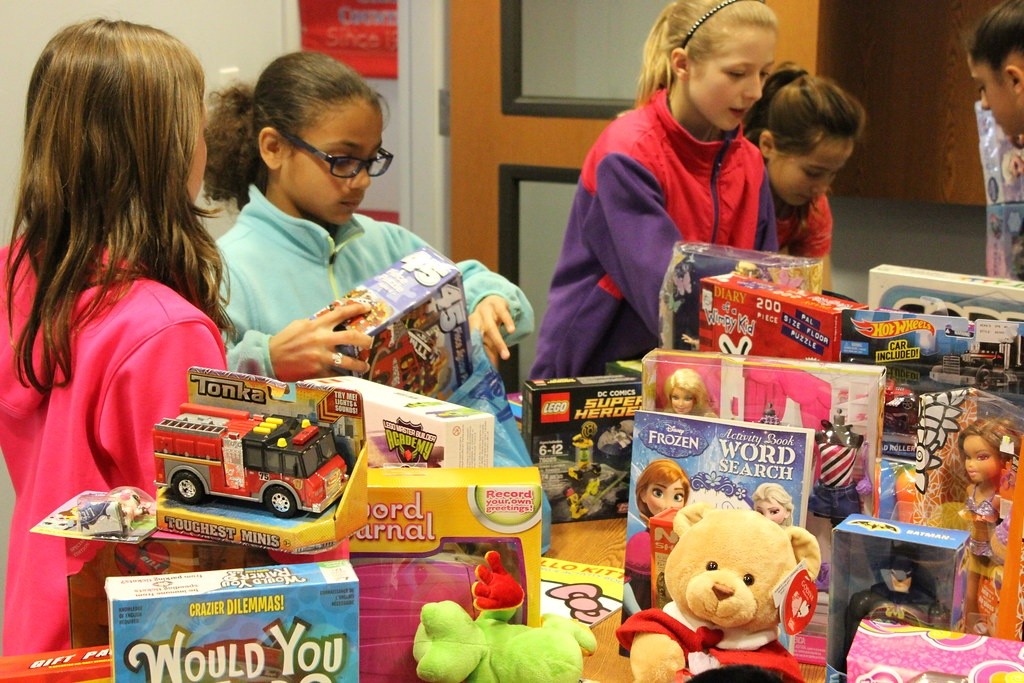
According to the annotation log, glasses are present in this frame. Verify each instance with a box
[270,124,394,178]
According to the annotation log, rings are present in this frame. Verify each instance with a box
[332,352,343,367]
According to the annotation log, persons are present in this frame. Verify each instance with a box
[838,540,951,675]
[662,369,718,420]
[744,61,866,293]
[806,422,864,526]
[201,52,534,382]
[530,1,779,376]
[0,17,232,641]
[957,415,1022,637]
[967,0,1024,138]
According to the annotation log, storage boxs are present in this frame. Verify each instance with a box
[0,243,1024,683]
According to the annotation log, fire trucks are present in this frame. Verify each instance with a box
[153,403,350,519]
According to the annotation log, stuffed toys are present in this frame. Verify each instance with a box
[413,551,597,683]
[618,498,821,683]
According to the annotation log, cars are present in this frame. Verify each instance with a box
[885,378,917,410]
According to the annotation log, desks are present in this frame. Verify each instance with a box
[538,512,829,683]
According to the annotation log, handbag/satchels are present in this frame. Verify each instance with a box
[439,330,554,562]
